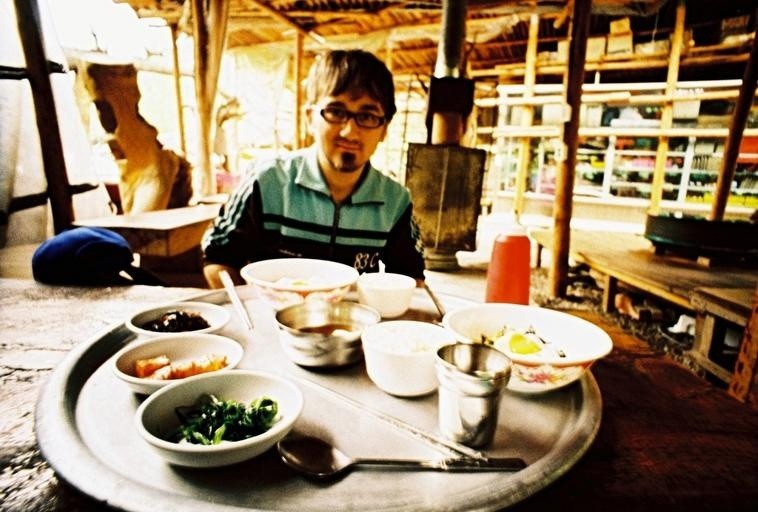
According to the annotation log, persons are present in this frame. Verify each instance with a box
[199,49,426,290]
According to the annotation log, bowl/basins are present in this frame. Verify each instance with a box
[271,300,382,365]
[237,258,361,306]
[136,369,302,465]
[126,301,224,335]
[442,302,615,394]
[114,336,243,394]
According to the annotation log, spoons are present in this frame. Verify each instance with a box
[276,435,530,486]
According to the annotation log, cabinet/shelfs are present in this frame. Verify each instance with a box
[492,126,758,216]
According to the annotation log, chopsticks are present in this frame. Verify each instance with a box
[216,268,256,331]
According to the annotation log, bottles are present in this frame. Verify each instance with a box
[485,233,536,308]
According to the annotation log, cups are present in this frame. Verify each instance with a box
[360,321,448,396]
[435,341,513,450]
[357,271,416,318]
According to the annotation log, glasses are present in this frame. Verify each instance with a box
[313,103,388,128]
